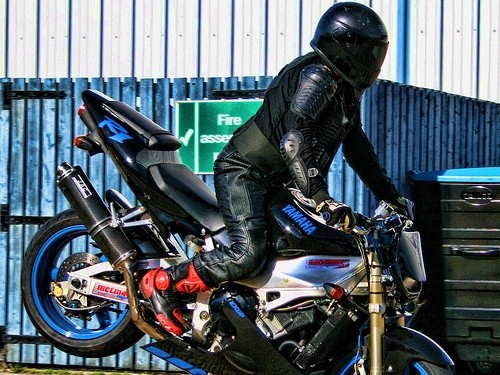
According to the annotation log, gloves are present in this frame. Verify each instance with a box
[397,196,419,224]
[315,194,356,231]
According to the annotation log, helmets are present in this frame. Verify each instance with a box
[309,1,391,89]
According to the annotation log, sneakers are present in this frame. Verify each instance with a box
[133,256,214,337]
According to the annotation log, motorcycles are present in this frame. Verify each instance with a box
[20,89,455,375]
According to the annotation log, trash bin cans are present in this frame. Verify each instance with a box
[408,166,500,375]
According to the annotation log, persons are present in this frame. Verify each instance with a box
[138,2,414,340]
